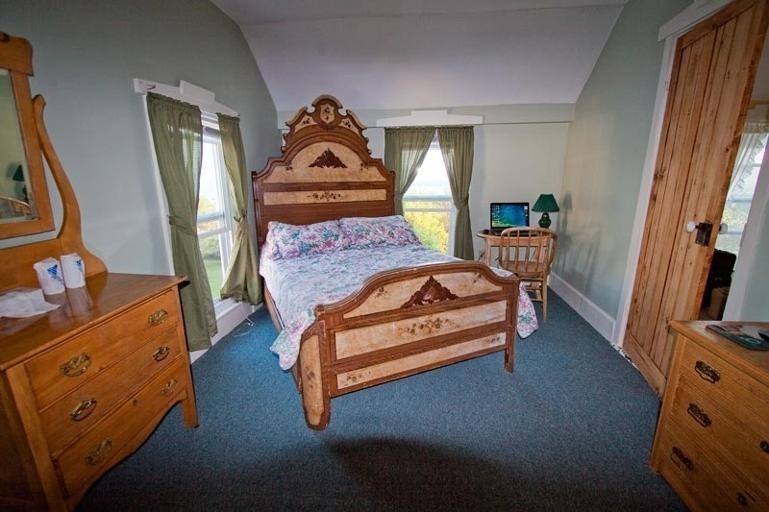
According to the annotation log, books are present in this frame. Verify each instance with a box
[706,324,768,350]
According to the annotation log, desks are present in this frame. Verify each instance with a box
[477,229,548,266]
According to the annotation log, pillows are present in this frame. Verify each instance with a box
[274,221,351,259]
[340,214,422,247]
[266,221,303,262]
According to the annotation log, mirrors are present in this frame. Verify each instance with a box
[0,31,55,240]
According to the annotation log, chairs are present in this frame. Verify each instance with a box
[495,227,557,323]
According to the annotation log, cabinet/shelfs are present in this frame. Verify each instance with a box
[650,320,769,511]
[0,271,199,512]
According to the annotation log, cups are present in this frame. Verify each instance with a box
[33,252,88,295]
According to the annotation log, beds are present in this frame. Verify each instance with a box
[251,95,519,431]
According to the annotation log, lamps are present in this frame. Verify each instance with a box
[532,193,559,229]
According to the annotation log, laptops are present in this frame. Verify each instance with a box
[491,203,537,236]
